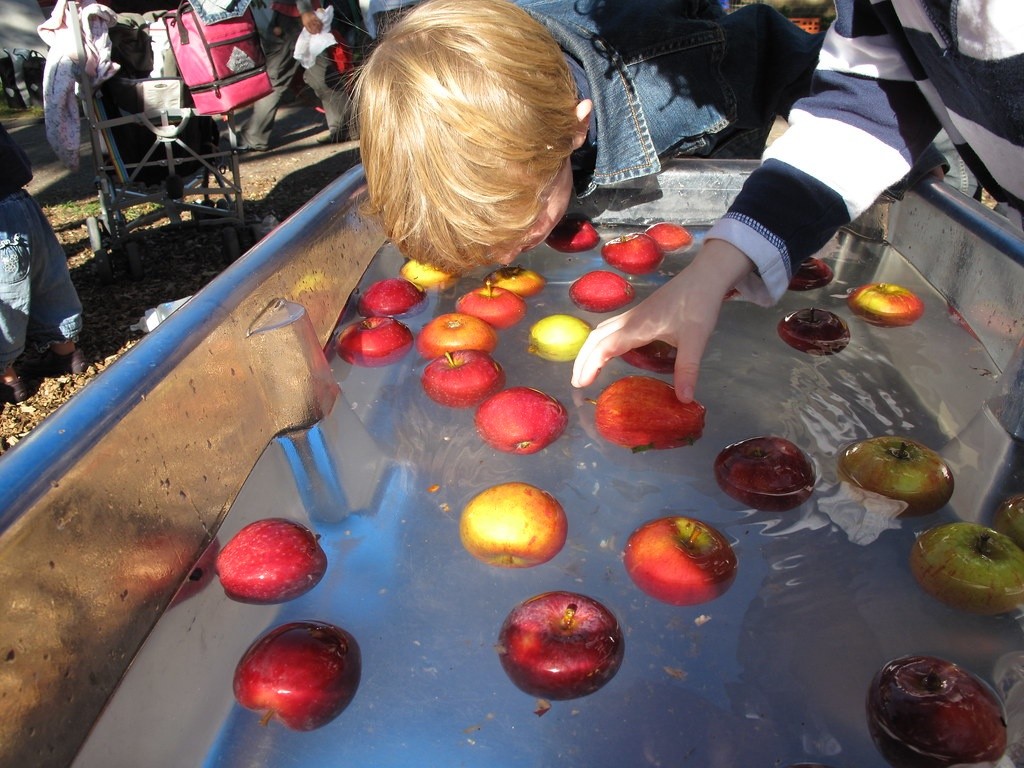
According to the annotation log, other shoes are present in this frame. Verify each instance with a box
[223,132,268,151]
[0,376,26,402]
[317,132,361,144]
[24,348,87,375]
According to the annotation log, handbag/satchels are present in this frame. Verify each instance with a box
[0,47,46,111]
[164,1,275,116]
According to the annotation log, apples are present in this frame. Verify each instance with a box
[866,654,1007,768]
[334,216,1024,615]
[497,590,625,700]
[231,619,361,733]
[212,517,328,605]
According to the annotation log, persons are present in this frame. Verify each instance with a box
[223,0,361,152]
[0,123,86,399]
[571,0,1024,402]
[355,0,952,280]
[319,0,425,66]
